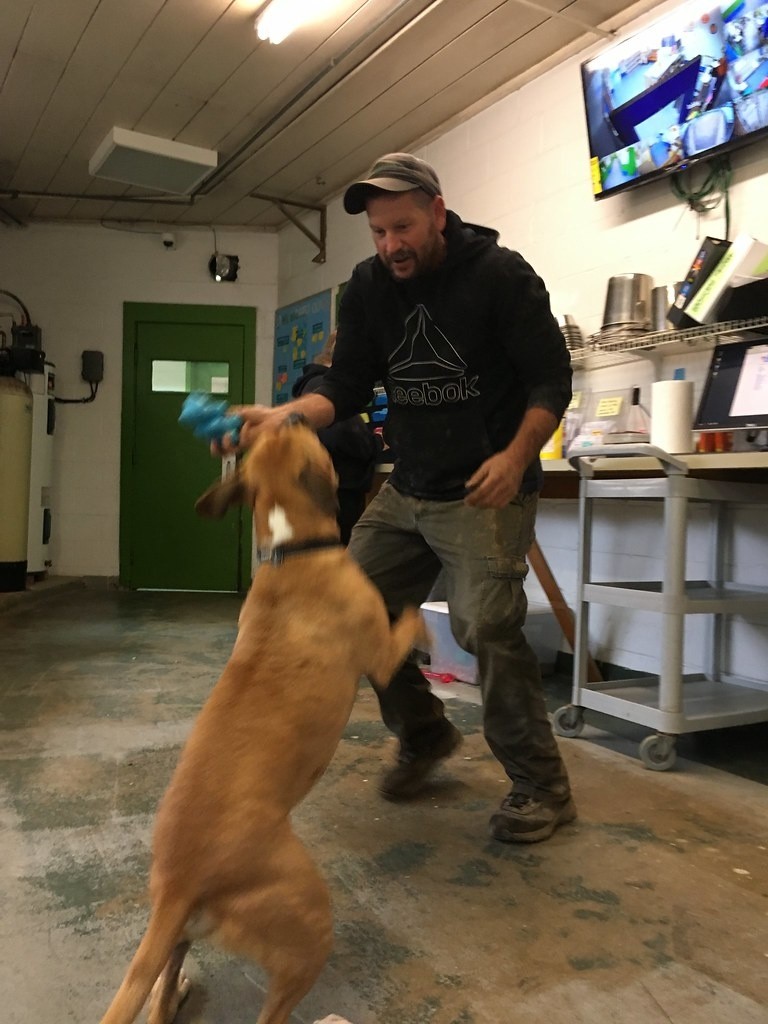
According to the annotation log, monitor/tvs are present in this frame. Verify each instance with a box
[580,0,768,203]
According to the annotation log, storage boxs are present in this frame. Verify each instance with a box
[420,602,562,685]
[668,236,768,330]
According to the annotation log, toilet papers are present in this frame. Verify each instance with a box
[650,379,696,455]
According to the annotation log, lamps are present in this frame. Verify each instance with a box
[87,126,218,197]
[254,0,343,45]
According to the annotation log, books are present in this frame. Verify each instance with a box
[666,235,768,329]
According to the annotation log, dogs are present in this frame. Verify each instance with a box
[98,409,433,1024]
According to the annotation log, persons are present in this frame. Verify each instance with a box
[208,152,577,843]
[294,331,388,545]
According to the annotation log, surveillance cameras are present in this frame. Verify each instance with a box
[162,233,174,247]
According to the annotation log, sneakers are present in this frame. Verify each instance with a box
[377,723,462,801]
[487,780,578,845]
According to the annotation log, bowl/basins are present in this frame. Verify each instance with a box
[554,314,584,351]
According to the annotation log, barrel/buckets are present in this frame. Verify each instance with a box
[599,272,655,347]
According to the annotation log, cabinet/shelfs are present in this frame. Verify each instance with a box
[553,443,768,772]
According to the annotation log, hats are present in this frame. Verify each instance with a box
[343,152,443,215]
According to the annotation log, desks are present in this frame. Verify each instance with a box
[372,453,768,500]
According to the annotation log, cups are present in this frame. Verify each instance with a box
[650,379,694,454]
